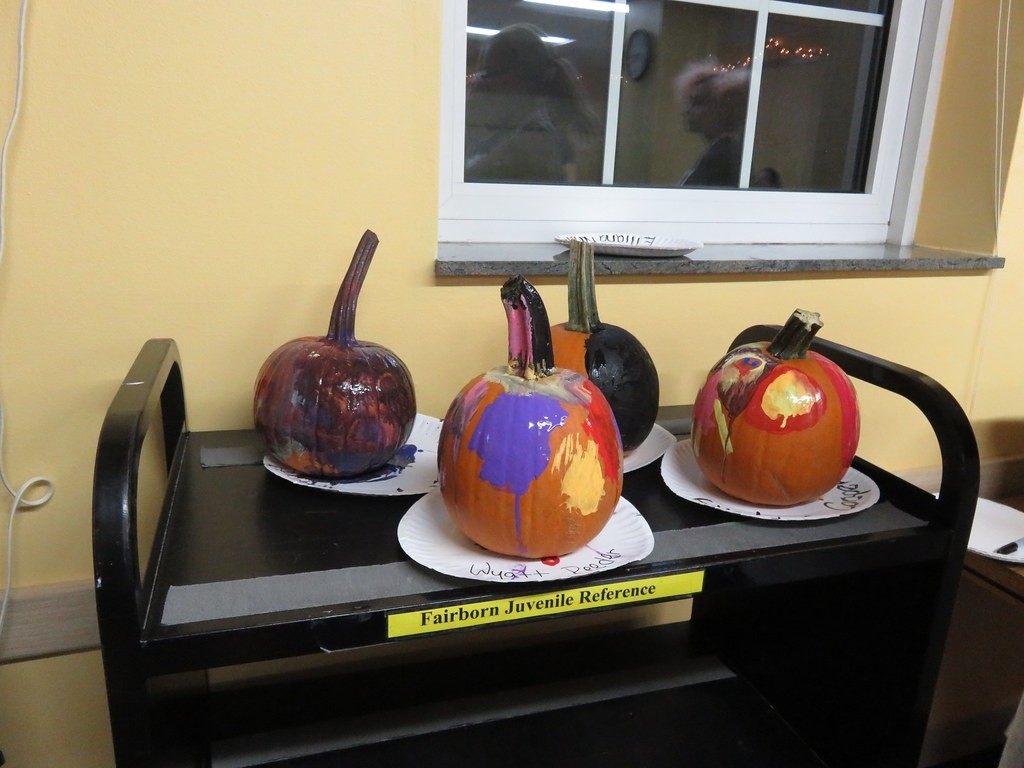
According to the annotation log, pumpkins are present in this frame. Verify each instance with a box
[250,229,861,560]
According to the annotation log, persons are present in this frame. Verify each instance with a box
[464,19,602,183]
[672,60,756,191]
[754,165,784,189]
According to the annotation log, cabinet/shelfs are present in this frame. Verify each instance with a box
[91,324,981,767]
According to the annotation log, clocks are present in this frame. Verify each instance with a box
[626,28,651,79]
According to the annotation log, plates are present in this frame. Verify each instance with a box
[661,438,880,521]
[933,491,1023,564]
[397,486,656,584]
[263,413,443,496]
[624,423,677,474]
